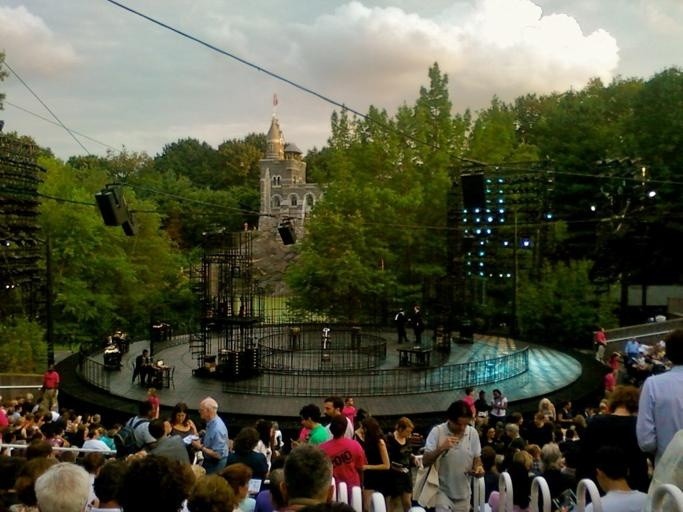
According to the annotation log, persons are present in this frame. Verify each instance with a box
[408,306,423,344]
[0,327,682,512]
[395,308,409,343]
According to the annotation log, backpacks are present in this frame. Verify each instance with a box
[113,417,151,452]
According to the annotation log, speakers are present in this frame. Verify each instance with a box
[462,236,473,250]
[122,216,136,236]
[461,171,486,208]
[97,188,130,225]
[278,222,297,245]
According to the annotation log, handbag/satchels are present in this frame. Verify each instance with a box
[411,463,439,508]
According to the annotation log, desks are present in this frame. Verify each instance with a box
[396,345,433,368]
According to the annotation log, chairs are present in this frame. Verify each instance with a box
[103,318,177,395]
